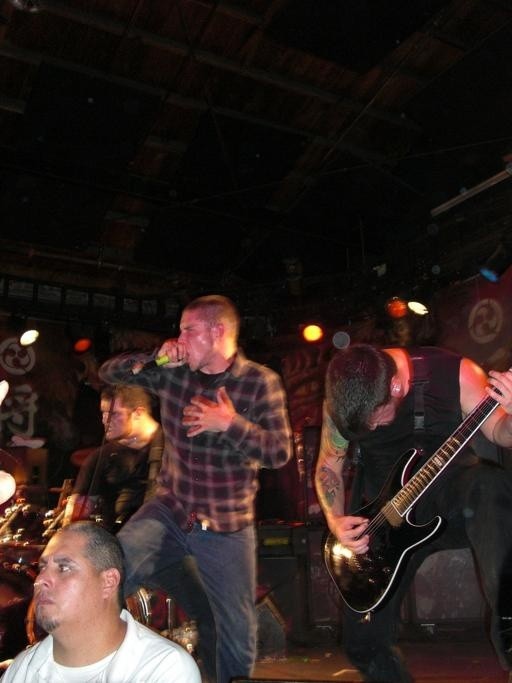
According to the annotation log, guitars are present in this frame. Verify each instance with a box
[325,365,512,615]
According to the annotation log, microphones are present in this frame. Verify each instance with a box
[139,354,171,372]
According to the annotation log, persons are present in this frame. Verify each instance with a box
[1,518,206,682]
[314,341,512,681]
[62,382,164,536]
[99,292,294,683]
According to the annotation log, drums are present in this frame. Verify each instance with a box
[124,586,184,635]
[0,502,58,590]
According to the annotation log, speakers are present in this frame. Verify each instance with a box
[253,556,310,645]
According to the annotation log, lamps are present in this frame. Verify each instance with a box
[14,316,93,355]
[386,236,511,319]
